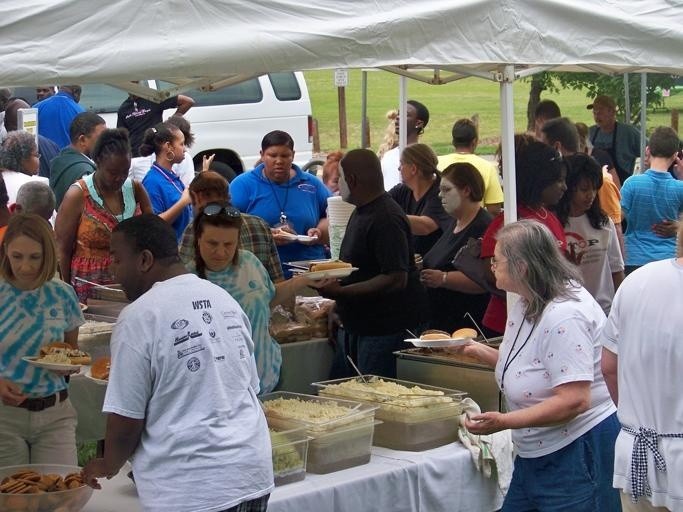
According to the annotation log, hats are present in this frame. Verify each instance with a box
[587,96,616,110]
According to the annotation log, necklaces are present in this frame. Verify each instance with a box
[497,303,536,412]
[93,174,124,224]
[268,178,290,225]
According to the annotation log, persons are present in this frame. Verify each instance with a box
[386,143,457,257]
[0,87,13,148]
[441,218,623,512]
[534,100,561,142]
[539,117,626,263]
[31,84,88,151]
[377,100,430,192]
[29,85,57,108]
[0,129,57,232]
[323,151,345,197]
[317,148,433,383]
[50,111,107,212]
[435,117,504,218]
[182,200,341,404]
[3,98,64,180]
[0,212,87,463]
[162,115,195,189]
[618,124,683,278]
[54,127,155,306]
[227,129,336,282]
[375,110,399,163]
[80,212,275,512]
[138,121,216,256]
[586,95,649,188]
[116,78,196,184]
[0,181,60,246]
[599,206,683,512]
[180,171,286,285]
[550,152,627,317]
[574,121,623,191]
[632,144,683,238]
[479,132,567,337]
[416,161,494,337]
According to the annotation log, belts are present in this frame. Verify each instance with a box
[18,388,68,411]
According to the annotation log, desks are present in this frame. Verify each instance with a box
[81,433,513,510]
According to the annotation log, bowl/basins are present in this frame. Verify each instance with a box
[0,463,97,512]
[93,283,129,302]
[256,390,384,488]
[87,298,128,316]
[310,373,468,452]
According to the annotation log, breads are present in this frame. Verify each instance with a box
[310,262,353,272]
[452,328,478,339]
[42,344,90,363]
[90,357,111,381]
[420,329,451,340]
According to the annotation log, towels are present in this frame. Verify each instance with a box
[458,399,514,498]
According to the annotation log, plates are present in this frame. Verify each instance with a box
[21,355,82,372]
[84,367,109,386]
[303,266,359,280]
[403,336,472,348]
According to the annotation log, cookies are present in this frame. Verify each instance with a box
[0,470,86,512]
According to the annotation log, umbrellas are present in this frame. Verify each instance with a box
[0,0,683,113]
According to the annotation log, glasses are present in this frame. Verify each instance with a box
[550,152,563,166]
[490,256,509,267]
[202,203,241,219]
[28,152,41,158]
[439,185,460,193]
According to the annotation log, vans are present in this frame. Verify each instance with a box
[8,71,319,184]
